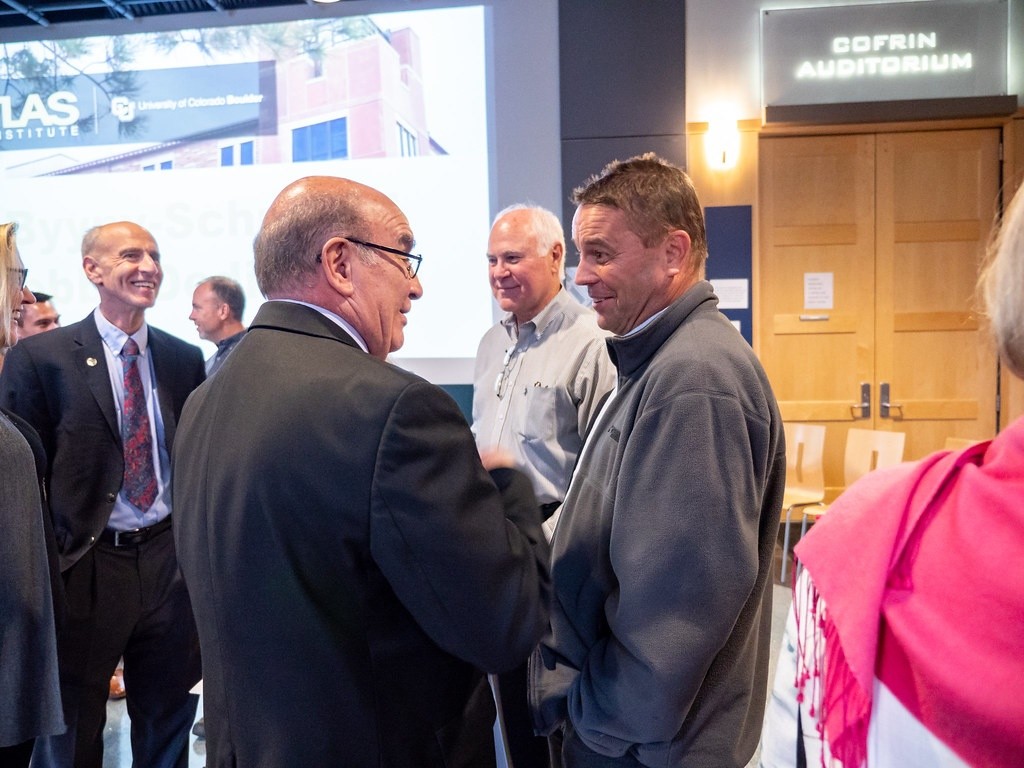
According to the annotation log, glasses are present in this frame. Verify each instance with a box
[7,268,27,291]
[316,237,421,279]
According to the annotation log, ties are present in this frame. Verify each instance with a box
[123,338,158,513]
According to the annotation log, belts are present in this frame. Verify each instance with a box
[102,516,172,547]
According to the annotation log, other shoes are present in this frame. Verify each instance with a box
[109,668,126,698]
[192,718,206,736]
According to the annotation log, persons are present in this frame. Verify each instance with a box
[0,150,787,768]
[771,183,1024,768]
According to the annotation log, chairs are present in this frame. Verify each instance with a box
[795,429,906,589]
[779,422,826,582]
